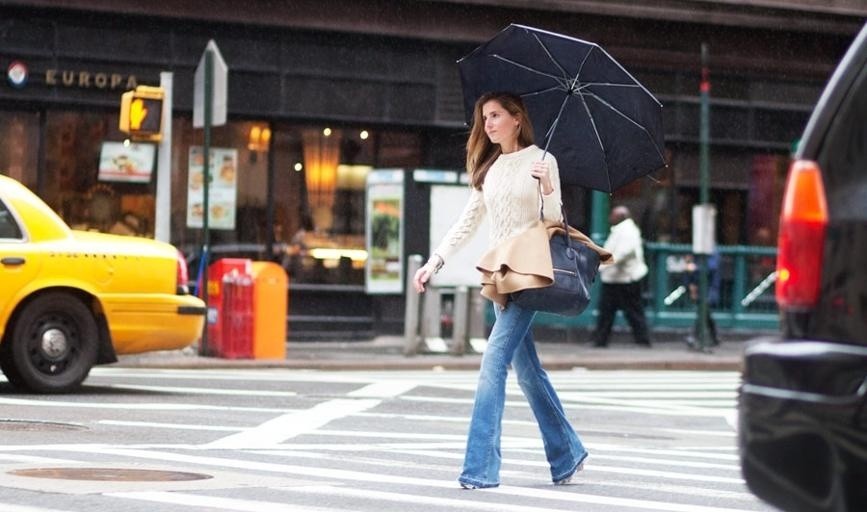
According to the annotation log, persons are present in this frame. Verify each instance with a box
[412,92,589,490]
[584,204,651,348]
[221,154,234,181]
[108,211,140,237]
[280,213,319,275]
[682,238,722,352]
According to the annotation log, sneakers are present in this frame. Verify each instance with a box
[554,459,586,486]
[460,482,480,491]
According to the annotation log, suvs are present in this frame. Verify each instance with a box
[729,7,866,512]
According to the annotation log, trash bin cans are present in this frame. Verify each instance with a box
[199,258,289,359]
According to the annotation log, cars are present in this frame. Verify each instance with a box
[0,169,212,397]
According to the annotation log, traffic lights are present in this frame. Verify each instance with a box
[126,85,166,137]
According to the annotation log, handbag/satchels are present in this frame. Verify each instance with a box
[509,202,602,317]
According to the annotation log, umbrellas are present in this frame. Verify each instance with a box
[456,21,667,200]
